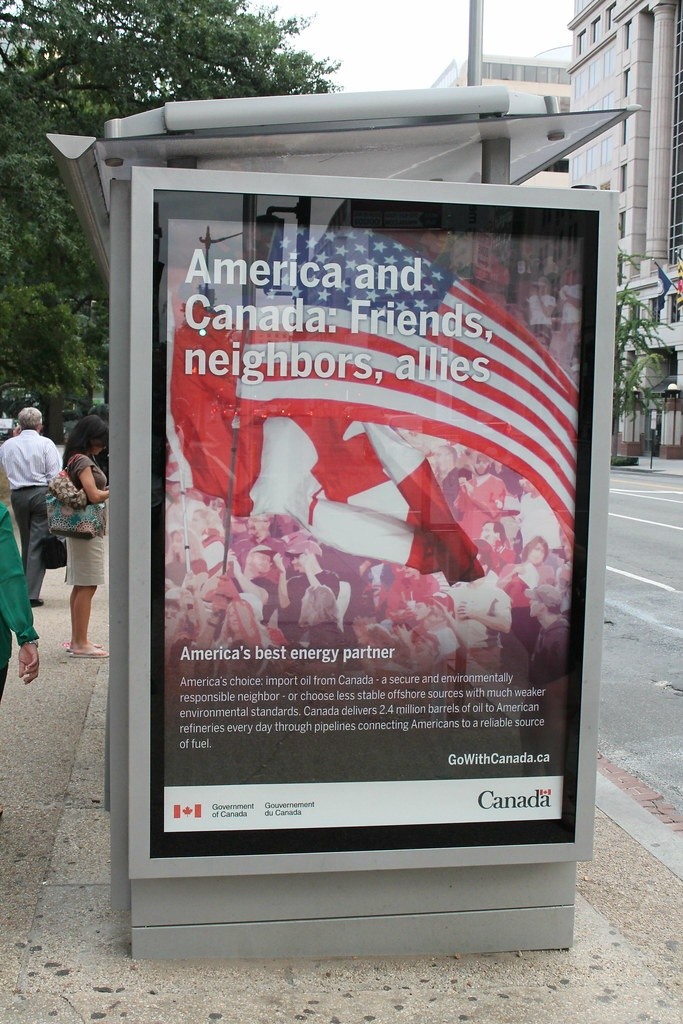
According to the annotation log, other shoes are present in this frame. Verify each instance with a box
[29,599,44,607]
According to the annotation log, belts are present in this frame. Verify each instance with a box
[10,484,48,494]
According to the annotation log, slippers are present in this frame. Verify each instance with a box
[69,647,110,658]
[66,643,104,652]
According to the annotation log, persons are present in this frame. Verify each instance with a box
[61,414,110,658]
[0,499,39,703]
[86,404,109,487]
[165,267,582,683]
[1,405,63,607]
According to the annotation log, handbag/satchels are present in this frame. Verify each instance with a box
[44,494,105,539]
[48,453,88,510]
[43,535,69,569]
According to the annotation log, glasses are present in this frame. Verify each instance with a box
[474,458,489,464]
[248,516,268,524]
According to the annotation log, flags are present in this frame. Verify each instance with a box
[676,255,683,312]
[239,209,577,555]
[658,266,674,314]
[172,293,486,584]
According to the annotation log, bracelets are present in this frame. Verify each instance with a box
[24,639,38,648]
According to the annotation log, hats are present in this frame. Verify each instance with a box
[228,591,263,621]
[525,584,563,608]
[284,539,321,558]
[166,588,196,608]
[250,539,287,557]
[512,561,540,589]
[420,592,454,612]
[166,470,192,488]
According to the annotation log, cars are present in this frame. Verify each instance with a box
[0,410,16,440]
